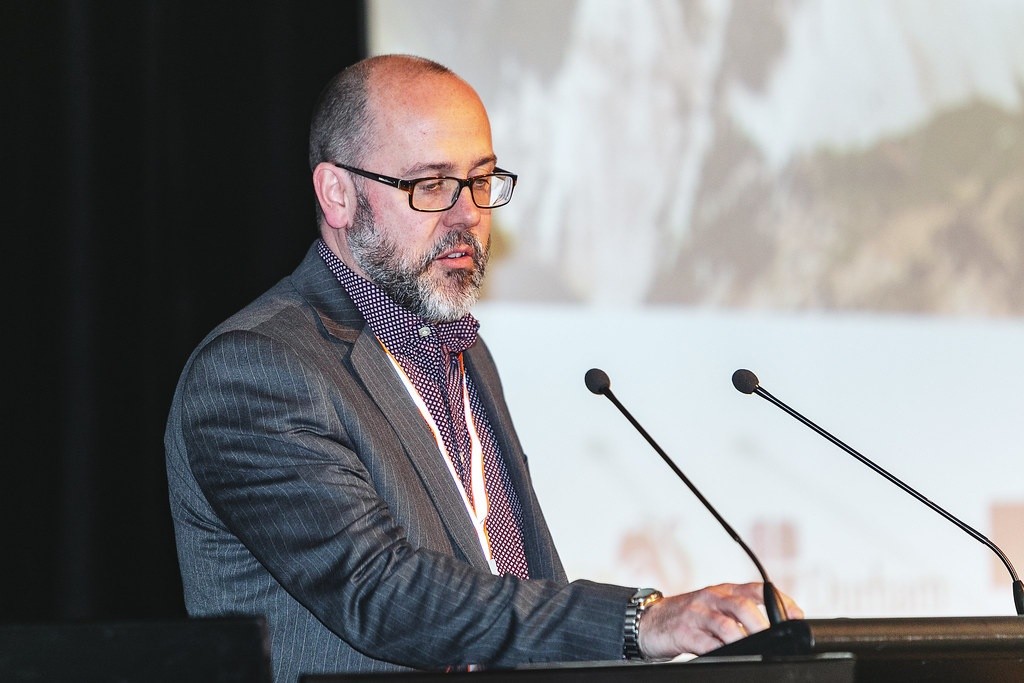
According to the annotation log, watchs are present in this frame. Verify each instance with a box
[623,587,662,661]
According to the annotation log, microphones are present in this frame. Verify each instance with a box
[732,370,1024,616]
[583,369,790,627]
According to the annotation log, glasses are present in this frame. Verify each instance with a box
[334,163,519,213]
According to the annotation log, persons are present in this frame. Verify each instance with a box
[162,55,804,683]
